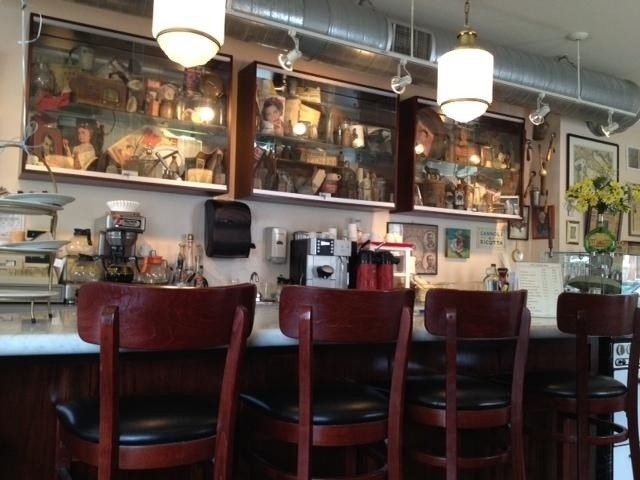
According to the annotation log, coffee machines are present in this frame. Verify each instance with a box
[93,213,148,284]
[287,238,351,289]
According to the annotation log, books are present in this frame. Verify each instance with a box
[33,114,61,166]
[58,112,99,171]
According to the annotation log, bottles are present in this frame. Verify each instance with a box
[173,235,209,288]
[356,249,395,291]
[485,264,499,292]
[334,125,352,148]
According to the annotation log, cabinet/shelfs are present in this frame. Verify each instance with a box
[389,97,525,221]
[237,61,399,211]
[20,12,231,199]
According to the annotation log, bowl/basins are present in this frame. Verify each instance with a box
[105,201,141,213]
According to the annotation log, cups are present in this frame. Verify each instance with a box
[159,100,175,119]
[327,223,403,243]
[78,44,95,71]
[325,172,342,182]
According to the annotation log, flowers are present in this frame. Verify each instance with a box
[564,166,640,214]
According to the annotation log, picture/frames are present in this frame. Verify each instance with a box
[565,133,620,200]
[386,222,439,275]
[566,219,581,246]
[532,205,556,240]
[628,188,640,237]
[506,205,530,240]
[585,202,623,245]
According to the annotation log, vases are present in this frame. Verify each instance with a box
[583,214,618,252]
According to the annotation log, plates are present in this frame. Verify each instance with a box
[0,192,77,210]
[1,289,60,299]
[0,239,71,254]
[141,145,186,178]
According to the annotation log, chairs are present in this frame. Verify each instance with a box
[237,283,412,479]
[50,280,257,479]
[524,291,640,480]
[385,289,535,479]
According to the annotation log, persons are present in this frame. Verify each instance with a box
[258,97,284,138]
[451,232,466,255]
[417,129,429,158]
[535,209,550,234]
[63,121,98,169]
[39,132,55,161]
[124,144,137,162]
[410,232,436,269]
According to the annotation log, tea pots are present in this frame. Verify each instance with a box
[141,250,170,284]
[65,227,92,254]
[70,252,100,283]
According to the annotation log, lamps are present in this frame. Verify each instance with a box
[437,2,495,127]
[151,0,227,68]
[275,20,621,138]
